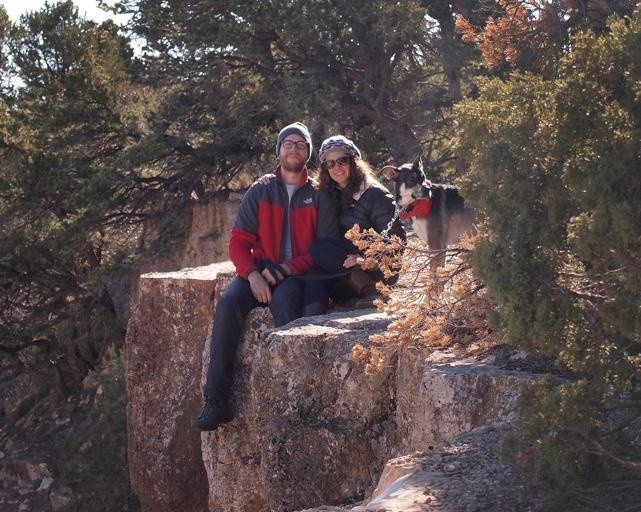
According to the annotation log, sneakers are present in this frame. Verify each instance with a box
[189,395,236,431]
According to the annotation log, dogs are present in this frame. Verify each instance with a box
[378,150,486,294]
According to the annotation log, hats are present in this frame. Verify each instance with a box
[319,134,361,170]
[275,122,313,164]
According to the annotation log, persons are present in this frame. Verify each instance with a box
[188,120,321,431]
[255,133,406,308]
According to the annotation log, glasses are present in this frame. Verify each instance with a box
[321,157,353,169]
[279,139,308,150]
